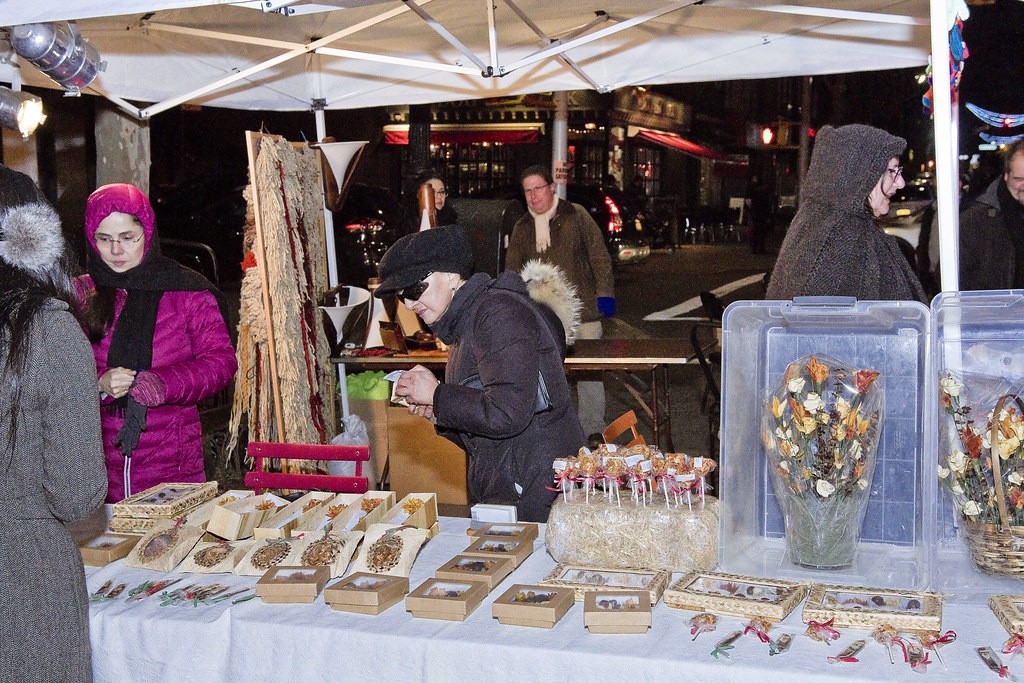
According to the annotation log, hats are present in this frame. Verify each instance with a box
[373,224,473,300]
[0,164,75,238]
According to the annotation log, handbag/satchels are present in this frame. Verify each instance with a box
[460,363,553,416]
[336,370,392,401]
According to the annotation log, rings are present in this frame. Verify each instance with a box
[124,386,128,392]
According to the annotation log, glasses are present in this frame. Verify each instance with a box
[434,191,449,197]
[94,230,144,248]
[888,167,903,184]
[525,183,549,195]
[396,270,433,304]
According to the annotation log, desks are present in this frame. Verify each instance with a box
[331,334,718,454]
[83,518,1024,681]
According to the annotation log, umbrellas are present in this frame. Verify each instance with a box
[1,0,971,543]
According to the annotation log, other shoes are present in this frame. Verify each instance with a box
[588,434,605,449]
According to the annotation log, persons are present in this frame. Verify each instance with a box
[624,174,649,245]
[398,169,458,240]
[506,164,615,322]
[934,137,1024,331]
[0,167,108,683]
[743,175,774,254]
[70,183,238,504]
[758,123,931,545]
[373,224,592,522]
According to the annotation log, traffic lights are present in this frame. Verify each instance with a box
[755,121,792,148]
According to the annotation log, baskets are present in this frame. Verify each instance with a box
[962,394,1024,580]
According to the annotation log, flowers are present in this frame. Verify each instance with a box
[761,354,880,501]
[938,372,1024,526]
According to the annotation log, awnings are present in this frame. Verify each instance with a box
[640,130,746,164]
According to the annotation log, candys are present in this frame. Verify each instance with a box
[553,443,717,510]
[688,613,1023,683]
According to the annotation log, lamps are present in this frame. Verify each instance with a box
[0,33,47,140]
[9,19,108,97]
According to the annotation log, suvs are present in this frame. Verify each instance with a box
[473,180,650,269]
[184,185,394,266]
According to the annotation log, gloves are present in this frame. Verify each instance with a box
[114,395,148,458]
[128,369,165,409]
[597,298,616,318]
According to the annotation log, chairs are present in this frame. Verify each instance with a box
[244,442,371,495]
[684,217,715,245]
[700,292,726,413]
[602,409,656,492]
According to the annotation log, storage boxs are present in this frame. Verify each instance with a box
[663,569,808,623]
[77,482,672,634]
[349,399,472,505]
[803,584,942,631]
[988,594,1024,637]
[716,289,1024,602]
